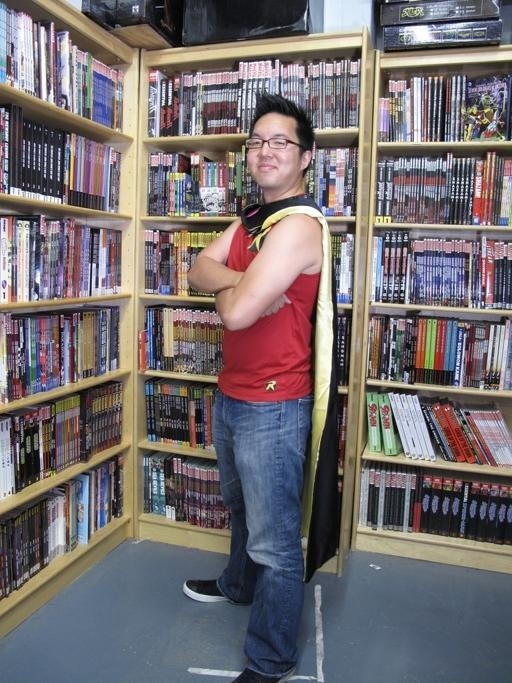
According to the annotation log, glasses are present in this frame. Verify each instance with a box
[245,137,306,152]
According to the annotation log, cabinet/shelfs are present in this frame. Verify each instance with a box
[140,26,375,577]
[350,45,512,576]
[0,0,139,635]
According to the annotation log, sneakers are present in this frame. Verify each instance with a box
[181,579,232,604]
[230,666,297,683]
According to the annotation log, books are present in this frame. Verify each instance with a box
[365,316,511,390]
[139,304,224,377]
[372,227,511,310]
[145,228,225,297]
[2,379,123,496]
[81,0,324,49]
[3,102,120,212]
[380,2,503,52]
[2,214,122,303]
[148,58,361,137]
[148,141,358,217]
[328,230,355,494]
[143,451,231,530]
[145,377,221,450]
[375,152,510,227]
[377,71,510,140]
[2,304,120,403]
[1,453,124,595]
[366,388,510,467]
[359,461,511,547]
[2,1,124,132]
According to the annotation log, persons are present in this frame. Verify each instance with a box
[180,87,331,683]
